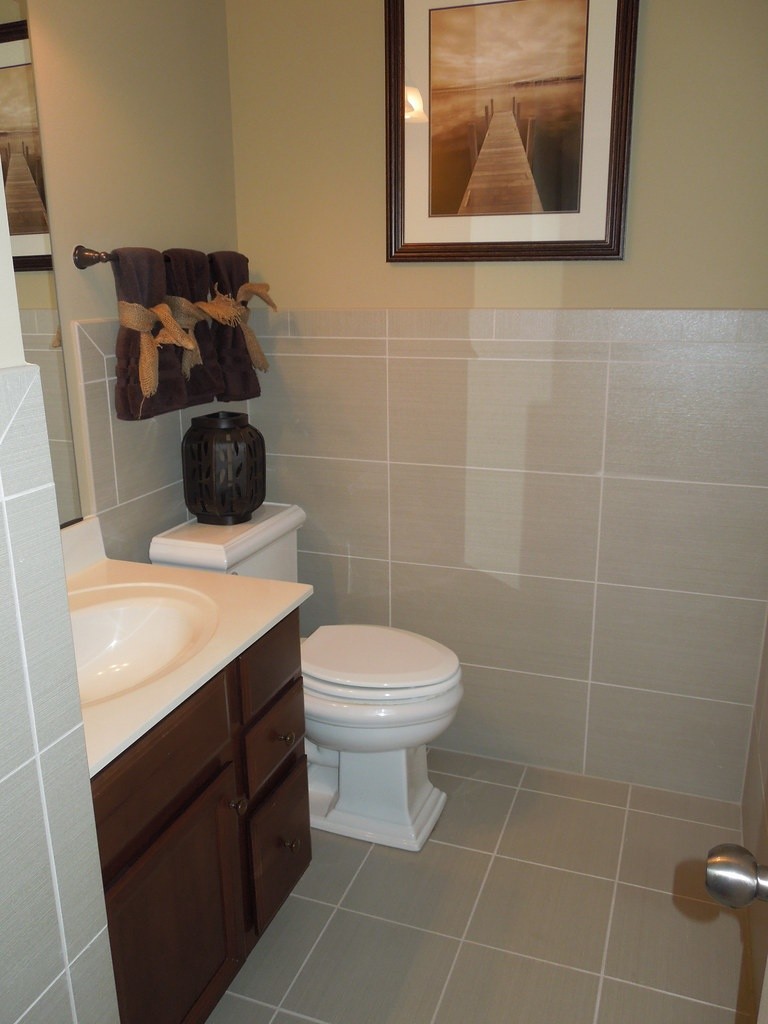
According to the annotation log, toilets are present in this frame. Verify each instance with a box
[149,502,464,852]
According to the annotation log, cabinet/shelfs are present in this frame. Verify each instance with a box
[89,670,246,1024]
[228,608,311,959]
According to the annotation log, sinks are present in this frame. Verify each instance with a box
[68,583,220,708]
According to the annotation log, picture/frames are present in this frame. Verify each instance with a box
[385,0,640,261]
[0,19,53,272]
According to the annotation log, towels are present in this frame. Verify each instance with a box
[207,251,262,402]
[162,248,226,409]
[111,247,189,422]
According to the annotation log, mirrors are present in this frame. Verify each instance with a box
[0,19,85,531]
